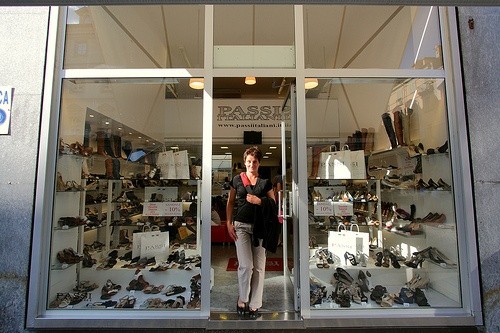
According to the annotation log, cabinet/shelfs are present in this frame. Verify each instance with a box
[46,107,462,311]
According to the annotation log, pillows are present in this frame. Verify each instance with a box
[211,210,221,223]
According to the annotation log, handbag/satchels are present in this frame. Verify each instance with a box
[327,223,356,261]
[333,145,367,180]
[157,145,176,179]
[174,146,190,179]
[140,225,169,259]
[318,145,343,179]
[132,225,160,259]
[341,224,369,261]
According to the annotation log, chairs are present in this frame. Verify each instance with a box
[211,221,234,248]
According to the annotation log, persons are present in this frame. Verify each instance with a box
[226,146,277,318]
[211,207,222,227]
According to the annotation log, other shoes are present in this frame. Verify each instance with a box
[48,131,201,309]
[233,162,242,172]
[236,299,246,317]
[222,177,231,189]
[249,308,258,319]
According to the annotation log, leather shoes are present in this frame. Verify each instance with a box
[307,111,457,307]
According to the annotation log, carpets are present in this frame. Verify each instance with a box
[226,257,294,271]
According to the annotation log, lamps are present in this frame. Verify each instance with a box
[189,78,204,90]
[245,77,256,85]
[305,78,318,89]
